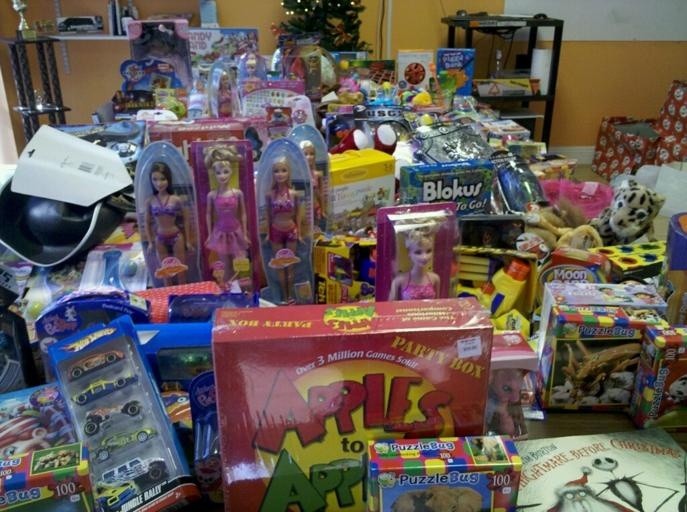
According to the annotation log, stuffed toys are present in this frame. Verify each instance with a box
[523,200,602,250]
[589,180,665,244]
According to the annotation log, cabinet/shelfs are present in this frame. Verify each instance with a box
[440,17,564,154]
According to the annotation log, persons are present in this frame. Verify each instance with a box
[218,72,233,116]
[263,159,305,302]
[389,231,439,301]
[299,140,326,226]
[143,162,194,285]
[245,58,261,81]
[202,144,253,278]
